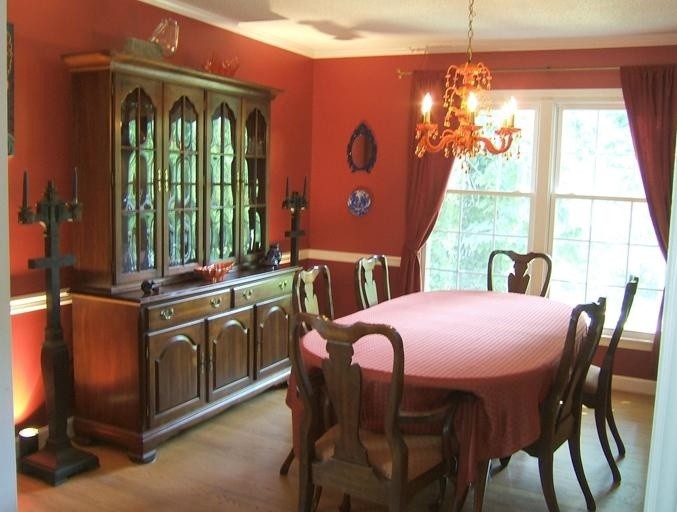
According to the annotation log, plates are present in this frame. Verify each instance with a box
[194,260,236,283]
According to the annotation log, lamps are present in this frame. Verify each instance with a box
[18,427,39,468]
[414,0,521,174]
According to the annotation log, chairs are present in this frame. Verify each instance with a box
[297,311,456,512]
[471,296,606,511]
[279,263,335,475]
[354,254,390,311]
[582,273,639,482]
[487,248,553,297]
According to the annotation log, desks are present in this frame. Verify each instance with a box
[297,288,587,511]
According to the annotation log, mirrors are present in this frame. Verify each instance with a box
[346,121,377,174]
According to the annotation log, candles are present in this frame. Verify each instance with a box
[303,176,307,195]
[21,168,29,209]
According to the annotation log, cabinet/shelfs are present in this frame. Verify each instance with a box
[254,271,297,379]
[204,71,270,271]
[147,289,255,429]
[112,53,205,284]
